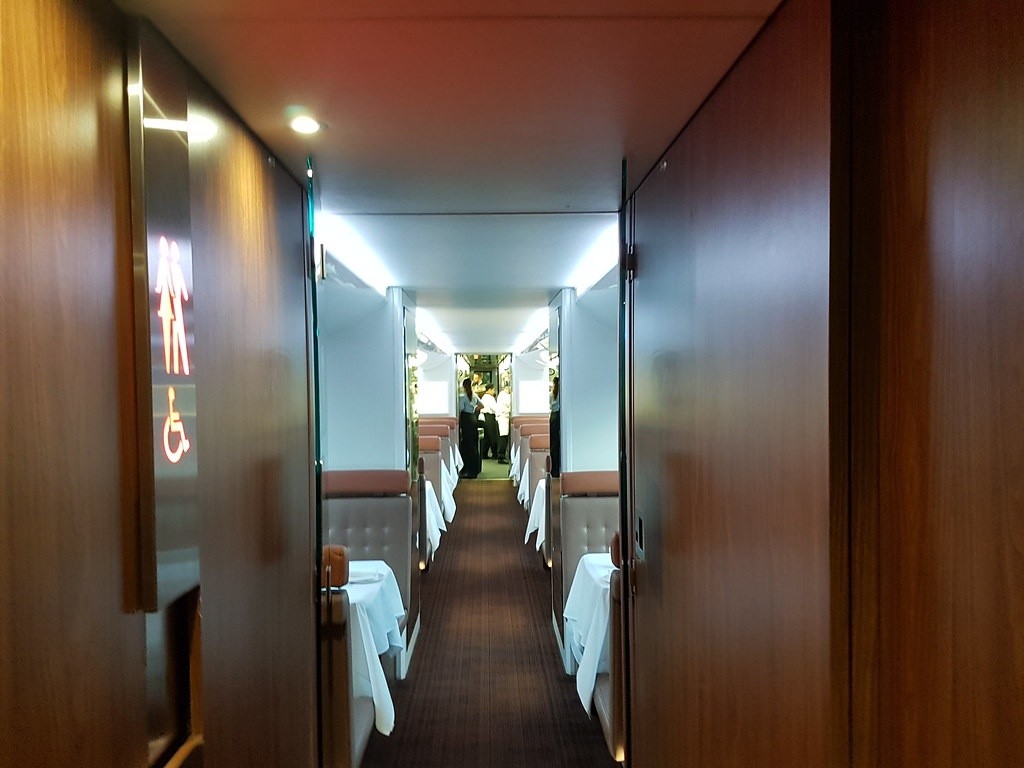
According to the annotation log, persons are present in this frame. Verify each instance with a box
[548,377,560,478]
[458,378,484,478]
[480,383,498,459]
[495,383,511,464]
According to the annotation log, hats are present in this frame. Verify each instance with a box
[486,383,494,387]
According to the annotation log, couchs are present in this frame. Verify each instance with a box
[329,409,624,768]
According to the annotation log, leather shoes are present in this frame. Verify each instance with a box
[458,455,509,478]
